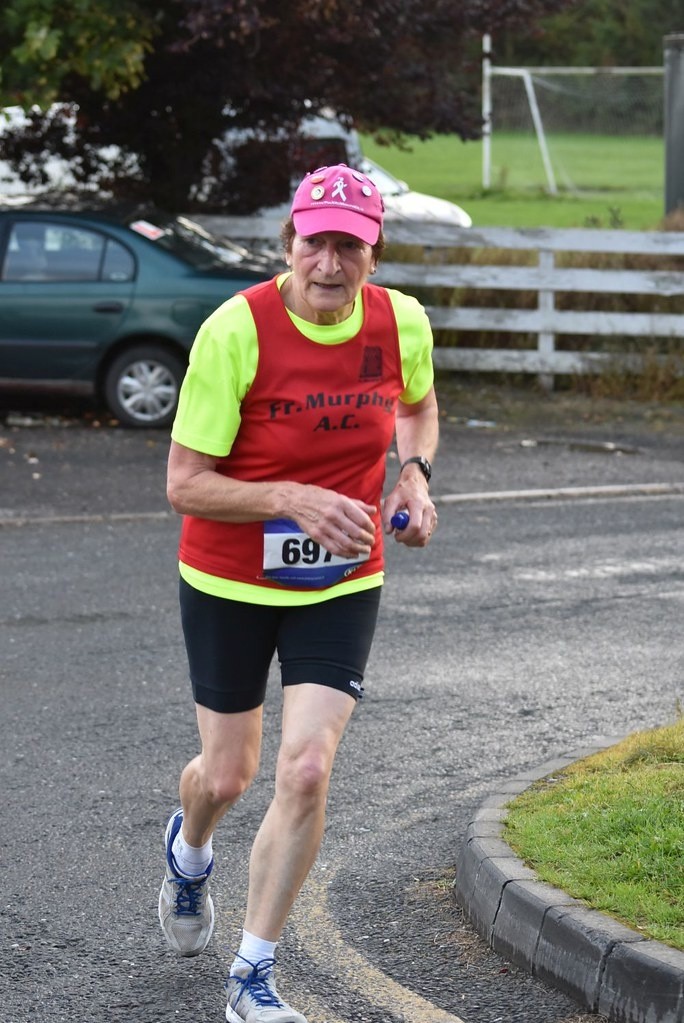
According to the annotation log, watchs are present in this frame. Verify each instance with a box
[400,456,432,482]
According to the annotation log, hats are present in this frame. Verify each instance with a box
[292,164,385,246]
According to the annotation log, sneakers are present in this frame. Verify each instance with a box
[157,807,215,957]
[226,969,310,1023]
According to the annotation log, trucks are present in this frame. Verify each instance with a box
[1,97,475,247]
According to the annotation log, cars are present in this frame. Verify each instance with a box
[0,196,291,429]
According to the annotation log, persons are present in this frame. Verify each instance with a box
[157,164,438,1023]
